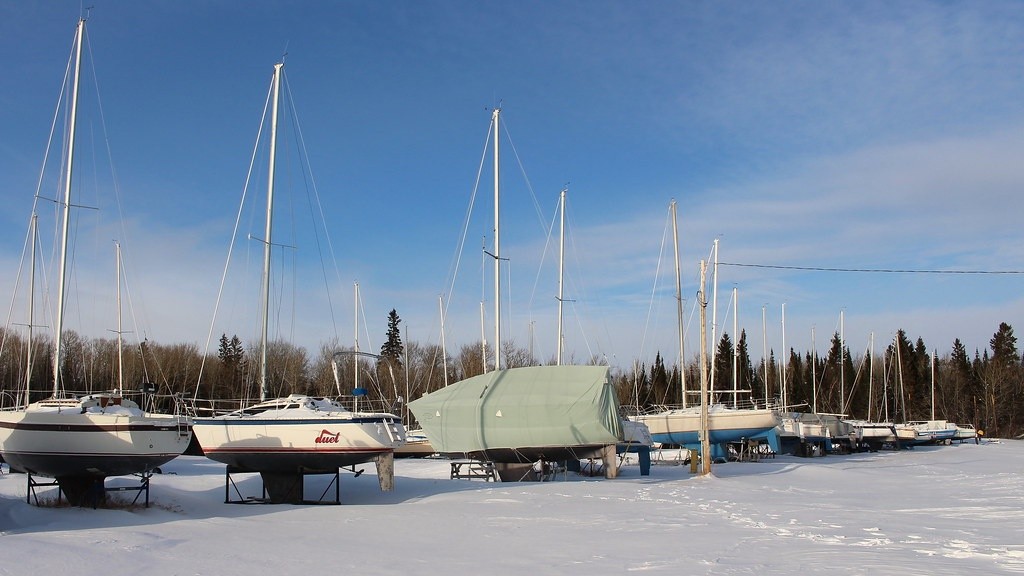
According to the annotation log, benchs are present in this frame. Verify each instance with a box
[450,461,496,483]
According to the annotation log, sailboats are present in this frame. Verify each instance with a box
[187,60,406,475]
[0,108,981,458]
[625,196,783,462]
[0,0,195,477]
